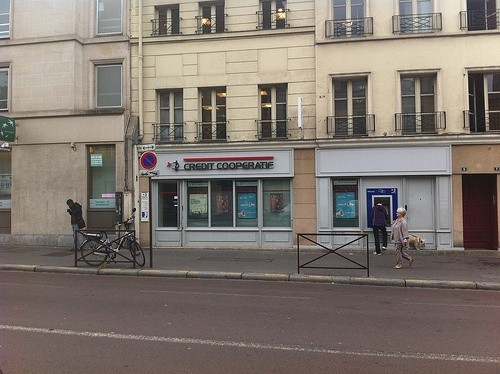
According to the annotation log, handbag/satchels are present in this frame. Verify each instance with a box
[77,219,85,229]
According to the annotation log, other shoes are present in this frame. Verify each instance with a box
[409,257,416,267]
[392,263,402,268]
[70,248,80,251]
[382,246,385,249]
[373,252,381,255]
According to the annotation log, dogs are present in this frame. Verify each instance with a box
[408,235,425,250]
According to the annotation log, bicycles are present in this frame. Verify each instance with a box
[80,215,146,267]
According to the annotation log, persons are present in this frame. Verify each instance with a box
[66,199,86,252]
[370,200,389,256]
[390,207,414,269]
